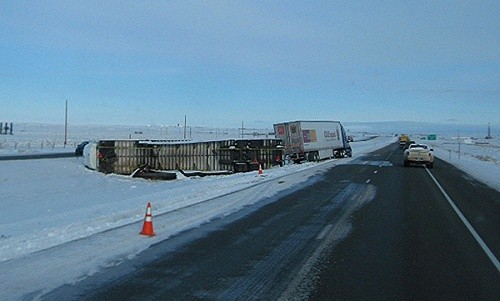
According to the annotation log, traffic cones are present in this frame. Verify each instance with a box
[258,164,263,174]
[139,202,157,237]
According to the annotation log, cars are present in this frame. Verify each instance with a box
[400,141,416,150]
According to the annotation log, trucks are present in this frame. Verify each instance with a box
[273,121,352,164]
[401,134,407,142]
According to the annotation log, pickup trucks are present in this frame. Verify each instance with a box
[403,144,435,167]
